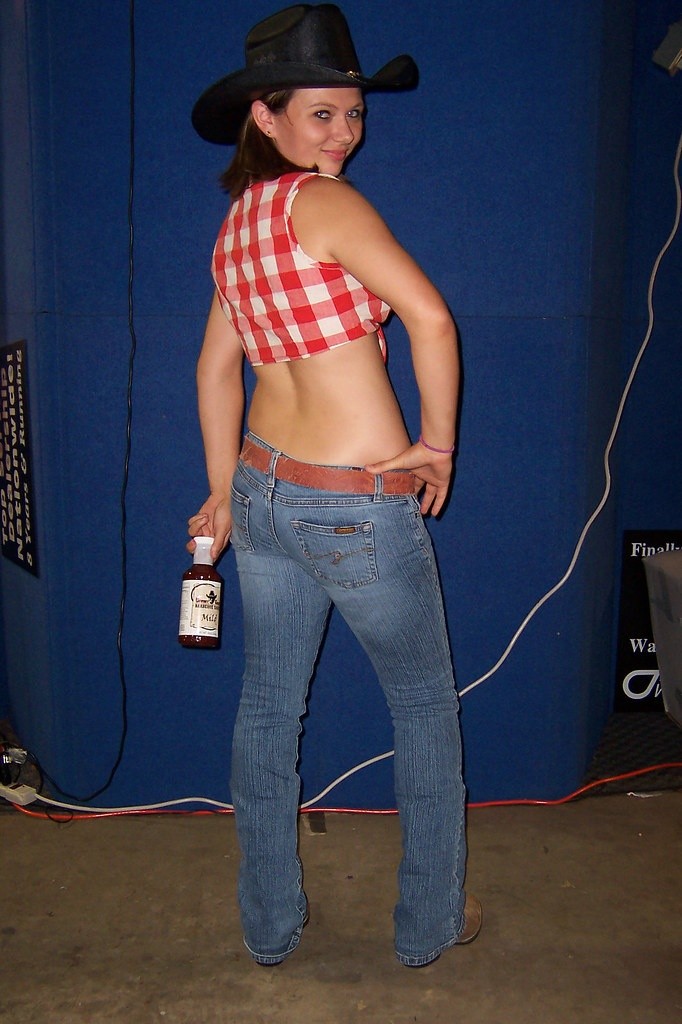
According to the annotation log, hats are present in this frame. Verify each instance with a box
[191,2,419,145]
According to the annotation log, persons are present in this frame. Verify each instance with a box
[187,3,512,967]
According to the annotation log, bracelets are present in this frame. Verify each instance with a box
[419,436,455,453]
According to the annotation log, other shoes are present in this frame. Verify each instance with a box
[453,896,482,944]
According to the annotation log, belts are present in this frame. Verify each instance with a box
[239,433,416,496]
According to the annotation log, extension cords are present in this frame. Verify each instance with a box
[0,781,38,807]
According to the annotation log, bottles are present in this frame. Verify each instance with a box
[179,535,223,649]
